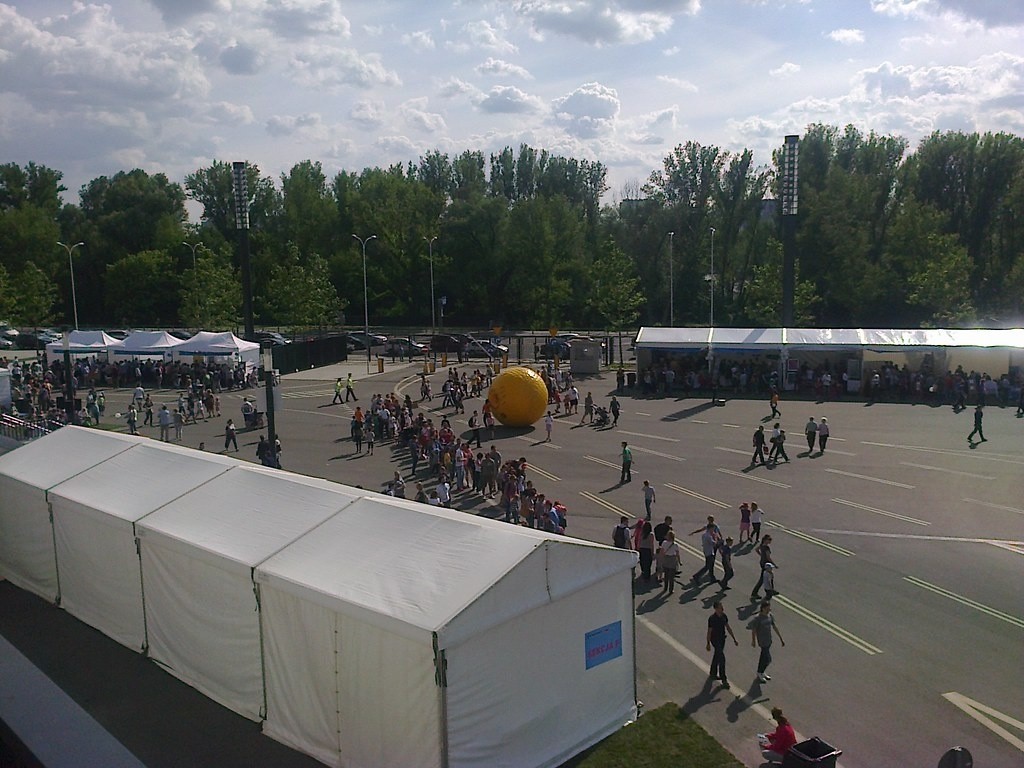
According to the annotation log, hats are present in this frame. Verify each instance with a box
[764,563,775,569]
[637,518,645,526]
[544,499,551,506]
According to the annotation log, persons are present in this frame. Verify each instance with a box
[759,708,797,764]
[751,534,779,601]
[617,368,625,394]
[944,364,1024,414]
[753,423,790,464]
[611,515,682,593]
[739,502,764,543]
[618,441,634,482]
[870,360,925,400]
[332,343,621,533]
[707,602,738,688]
[712,359,781,418]
[644,360,712,398]
[805,417,829,453]
[752,602,785,682]
[967,405,988,442]
[0,351,282,469]
[641,480,656,520]
[801,359,847,404]
[689,515,735,590]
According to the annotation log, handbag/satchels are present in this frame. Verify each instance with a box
[763,444,769,455]
[658,549,665,565]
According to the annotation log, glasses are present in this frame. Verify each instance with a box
[765,538,771,541]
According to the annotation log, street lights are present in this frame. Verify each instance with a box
[55,241,85,330]
[351,233,377,360]
[182,240,204,320]
[710,227,718,328]
[668,232,675,327]
[421,235,439,333]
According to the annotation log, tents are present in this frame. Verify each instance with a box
[177,331,260,374]
[107,331,185,366]
[0,425,643,768]
[46,330,124,370]
[636,327,1024,388]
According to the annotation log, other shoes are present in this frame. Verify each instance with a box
[718,581,731,590]
[756,539,760,542]
[982,439,987,442]
[739,540,743,544]
[640,569,717,593]
[772,590,779,595]
[967,437,973,441]
[750,594,761,599]
[756,672,771,683]
[710,673,719,680]
[749,537,753,542]
[747,537,750,540]
[722,681,730,689]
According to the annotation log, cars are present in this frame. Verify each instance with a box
[242,328,292,353]
[0,324,66,350]
[304,329,509,359]
[168,330,192,340]
[106,329,130,341]
[540,333,606,360]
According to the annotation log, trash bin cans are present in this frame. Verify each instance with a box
[790,735,843,768]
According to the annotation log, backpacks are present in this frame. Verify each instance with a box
[614,526,627,548]
